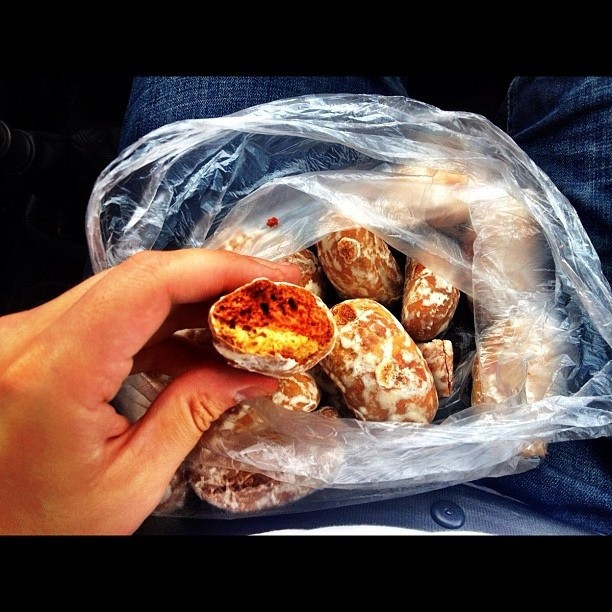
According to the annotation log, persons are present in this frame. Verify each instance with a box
[0,74,612,535]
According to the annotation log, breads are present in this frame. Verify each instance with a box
[190,405,351,514]
[464,202,539,319]
[473,320,557,461]
[212,227,322,297]
[319,298,439,425]
[265,372,321,414]
[317,206,402,306]
[208,277,335,377]
[152,464,185,517]
[359,165,483,231]
[417,339,454,398]
[402,257,462,341]
